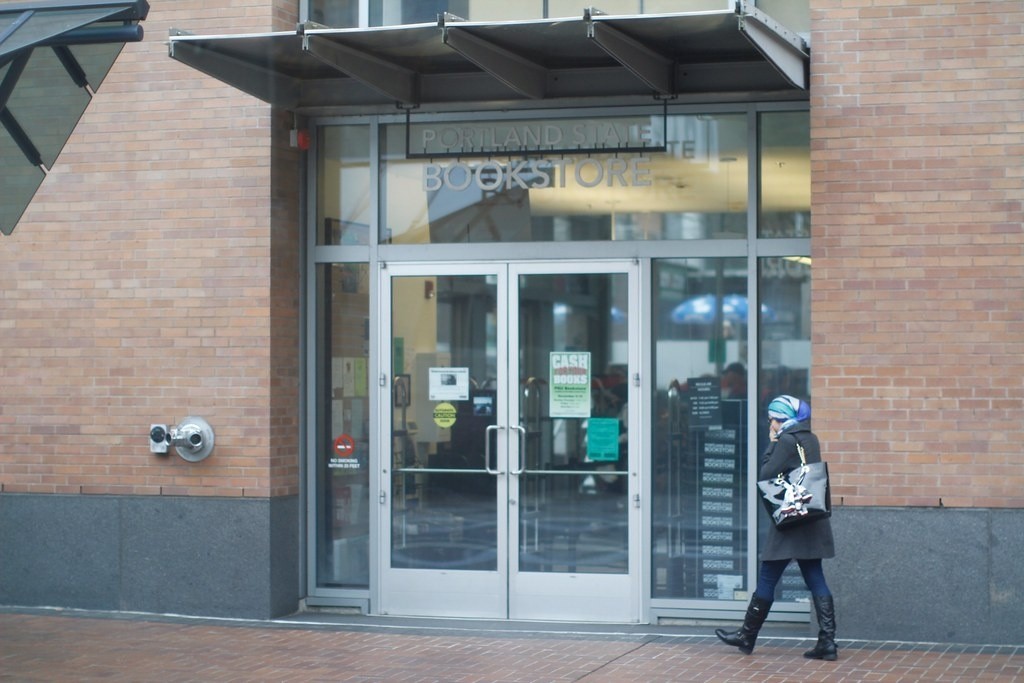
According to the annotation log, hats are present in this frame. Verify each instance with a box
[720,362,745,376]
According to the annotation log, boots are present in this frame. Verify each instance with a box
[802,595,839,661]
[715,592,774,656]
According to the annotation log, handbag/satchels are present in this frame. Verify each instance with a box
[757,444,832,528]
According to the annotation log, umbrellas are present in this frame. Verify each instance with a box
[671,293,776,324]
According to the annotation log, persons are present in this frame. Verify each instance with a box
[725,362,749,400]
[715,394,839,661]
[599,384,629,493]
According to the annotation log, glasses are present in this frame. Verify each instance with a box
[768,417,774,424]
